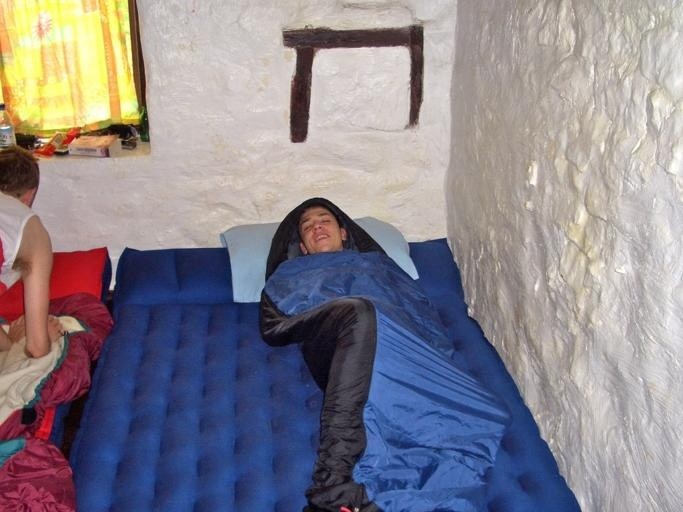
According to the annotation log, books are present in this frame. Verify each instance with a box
[68,134,123,158]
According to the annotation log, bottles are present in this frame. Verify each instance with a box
[0,103,15,150]
[138,108,148,142]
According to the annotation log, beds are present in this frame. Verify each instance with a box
[71,237,581,512]
[0,245,113,466]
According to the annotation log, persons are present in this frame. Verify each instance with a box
[295,205,348,256]
[1,144,61,359]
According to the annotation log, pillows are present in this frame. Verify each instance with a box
[221,218,421,303]
